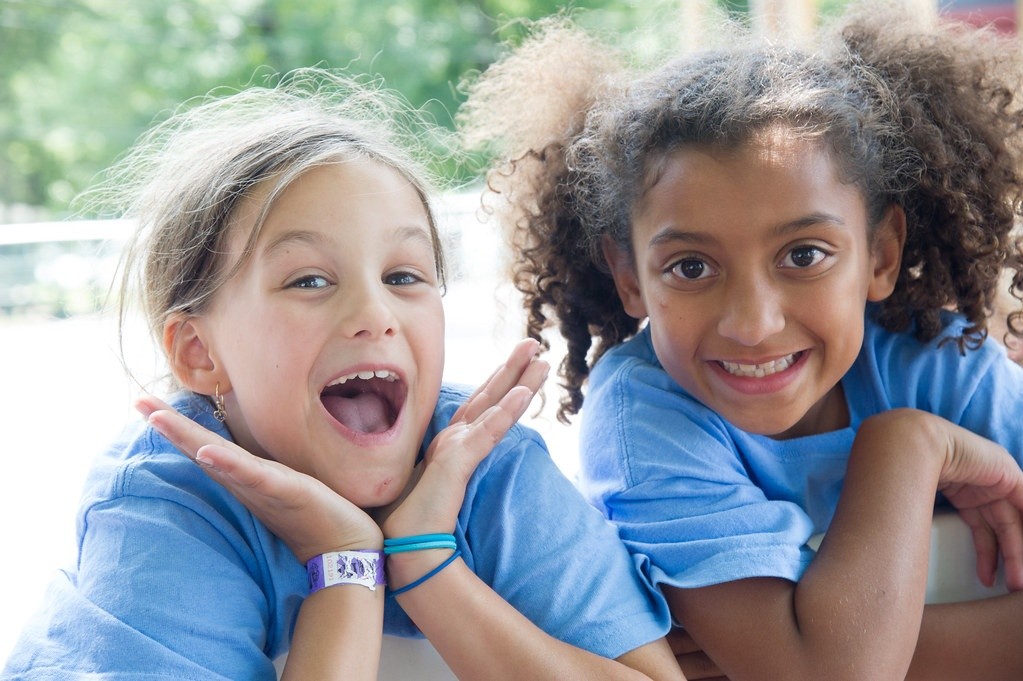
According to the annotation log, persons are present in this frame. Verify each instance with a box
[457,11,1023,681]
[0,85,697,679]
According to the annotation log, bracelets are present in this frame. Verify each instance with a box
[380,527,464,597]
[302,545,391,592]
